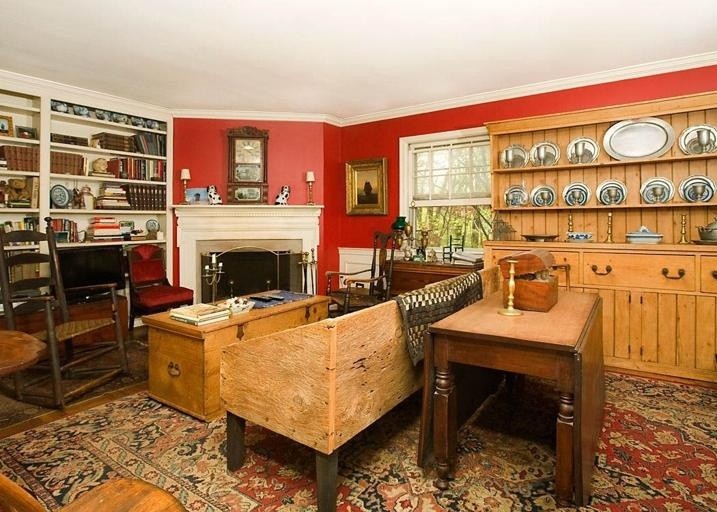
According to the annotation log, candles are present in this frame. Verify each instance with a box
[219,262,223,267]
[205,265,209,269]
[211,253,216,265]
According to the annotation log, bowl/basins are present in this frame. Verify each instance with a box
[566,231,593,239]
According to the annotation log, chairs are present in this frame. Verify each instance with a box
[326,230,398,318]
[127,244,193,331]
[0,217,130,409]
[0,472,185,512]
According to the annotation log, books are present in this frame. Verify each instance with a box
[86,217,125,242]
[133,134,166,156]
[0,145,40,172]
[169,303,231,326]
[95,182,131,210]
[50,133,88,147]
[92,132,135,152]
[0,216,39,246]
[4,249,39,284]
[107,157,166,182]
[452,258,484,267]
[451,251,484,262]
[50,151,89,176]
[218,296,255,315]
[120,184,166,210]
[25,176,39,209]
[50,218,78,243]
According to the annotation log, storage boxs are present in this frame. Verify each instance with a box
[498,248,559,312]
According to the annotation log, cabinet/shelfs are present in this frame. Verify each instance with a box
[0,69,173,312]
[483,90,717,390]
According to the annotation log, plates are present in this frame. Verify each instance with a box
[691,239,717,245]
[50,185,70,207]
[146,220,160,234]
[566,239,594,242]
[499,117,717,207]
[520,234,560,242]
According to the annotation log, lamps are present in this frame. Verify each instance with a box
[181,169,191,205]
[305,171,316,206]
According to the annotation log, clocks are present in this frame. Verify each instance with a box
[227,126,269,205]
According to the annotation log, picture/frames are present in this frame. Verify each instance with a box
[0,115,13,137]
[186,188,209,205]
[345,158,388,215]
[15,125,37,140]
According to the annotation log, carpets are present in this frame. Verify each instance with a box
[0,367,717,512]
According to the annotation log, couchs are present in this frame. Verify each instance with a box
[219,266,499,512]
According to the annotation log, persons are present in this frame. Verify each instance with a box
[194,193,200,201]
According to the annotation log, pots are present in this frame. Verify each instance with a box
[697,216,717,240]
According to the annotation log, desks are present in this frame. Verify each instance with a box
[386,258,483,297]
[417,285,603,510]
[142,289,333,424]
